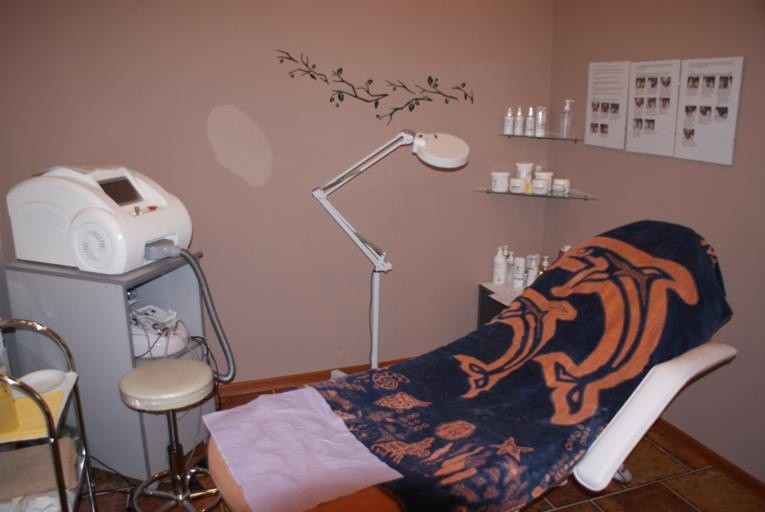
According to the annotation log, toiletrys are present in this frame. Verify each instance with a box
[488,99,574,292]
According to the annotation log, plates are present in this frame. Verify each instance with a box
[9,368,67,400]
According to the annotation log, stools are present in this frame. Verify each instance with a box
[119,359,225,512]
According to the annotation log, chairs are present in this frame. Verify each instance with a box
[207,219,737,511]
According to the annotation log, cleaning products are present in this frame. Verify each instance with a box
[502,105,550,138]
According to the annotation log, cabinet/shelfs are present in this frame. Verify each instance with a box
[0,318,97,511]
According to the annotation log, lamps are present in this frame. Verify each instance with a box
[310,127,471,370]
